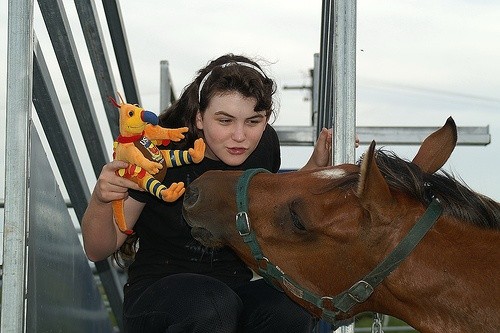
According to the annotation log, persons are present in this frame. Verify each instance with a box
[81,53,359,333]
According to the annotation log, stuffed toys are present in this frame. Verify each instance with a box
[107,91,206,235]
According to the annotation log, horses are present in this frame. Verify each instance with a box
[181,116,500,333]
